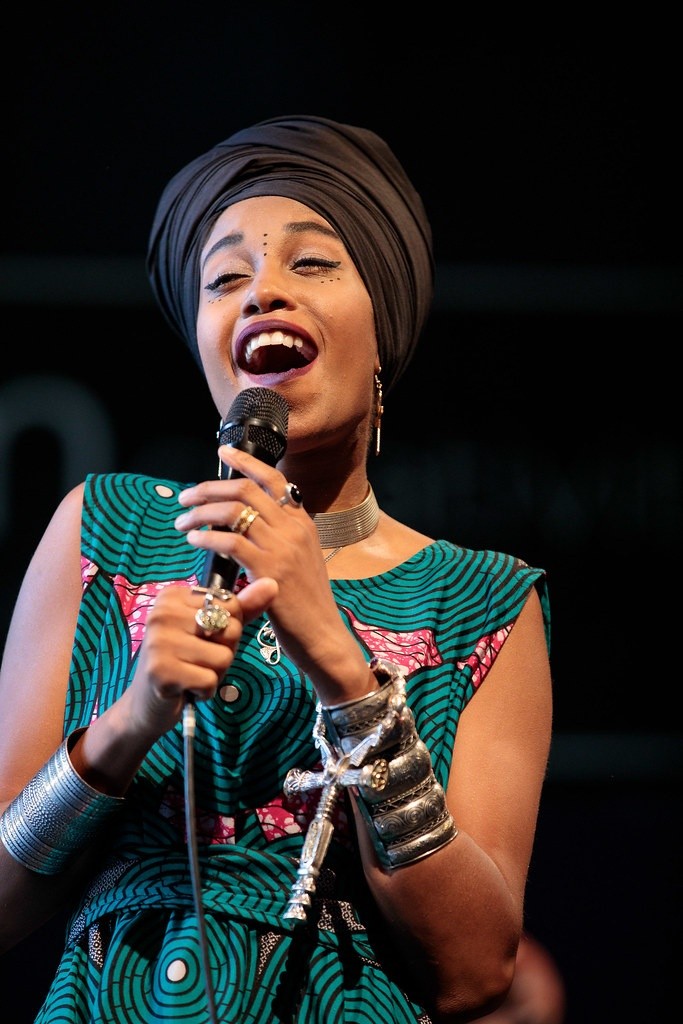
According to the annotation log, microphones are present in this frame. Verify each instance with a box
[199,387,291,590]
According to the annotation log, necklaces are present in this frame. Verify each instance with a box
[308,480,380,564]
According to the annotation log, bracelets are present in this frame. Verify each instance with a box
[0,726,128,877]
[282,663,459,921]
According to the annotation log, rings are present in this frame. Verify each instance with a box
[275,481,303,506]
[230,505,259,535]
[191,573,232,639]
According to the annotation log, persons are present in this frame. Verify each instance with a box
[0,112,556,1024]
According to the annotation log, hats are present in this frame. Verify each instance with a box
[143,115,435,401]
